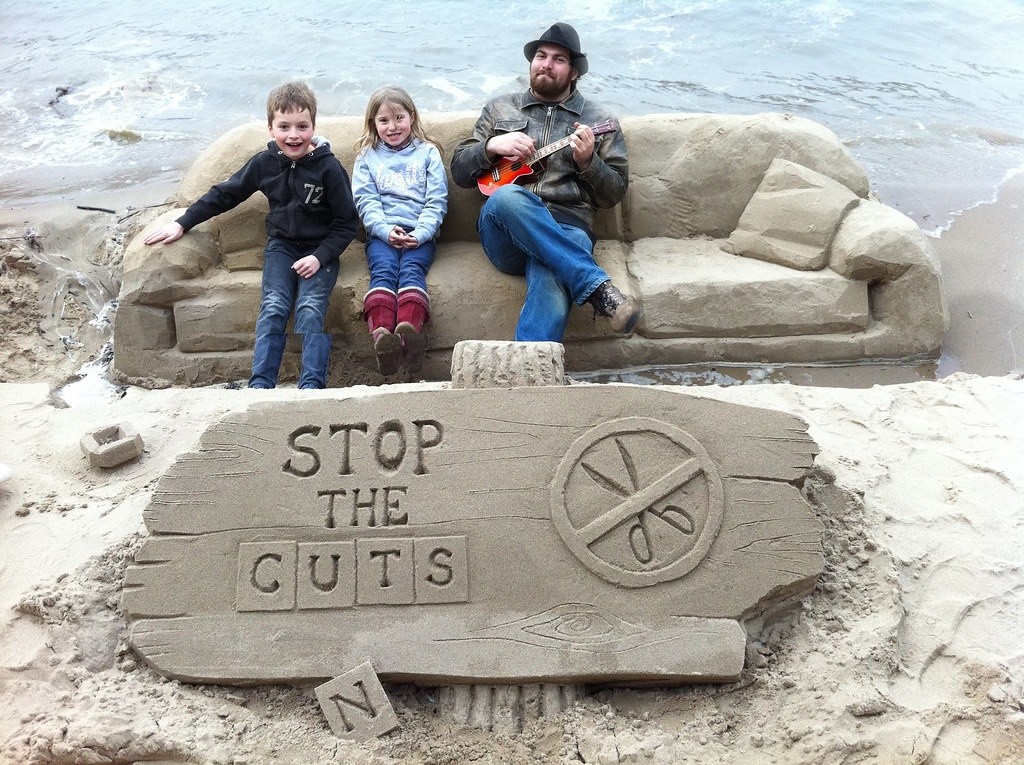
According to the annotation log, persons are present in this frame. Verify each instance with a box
[352,86,449,375]
[142,79,361,390]
[450,22,646,344]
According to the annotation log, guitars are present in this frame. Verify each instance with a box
[476,118,617,200]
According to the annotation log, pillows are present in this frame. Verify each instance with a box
[723,157,862,278]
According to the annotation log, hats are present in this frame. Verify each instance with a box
[524,22,588,78]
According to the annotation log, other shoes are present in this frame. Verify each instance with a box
[372,327,398,376]
[395,321,423,373]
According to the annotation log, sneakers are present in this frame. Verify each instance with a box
[587,280,644,335]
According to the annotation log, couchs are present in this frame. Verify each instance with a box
[114,114,945,377]
